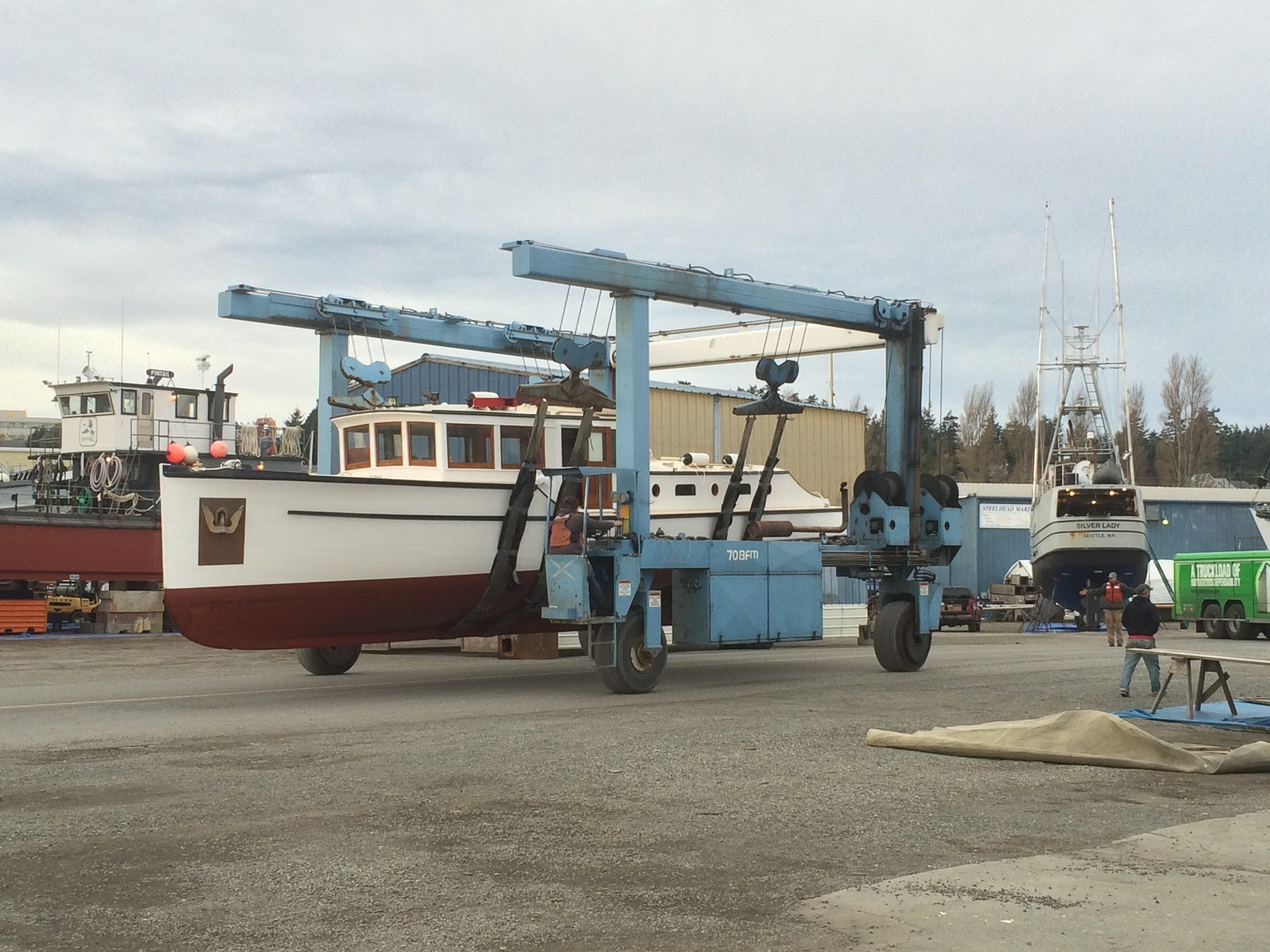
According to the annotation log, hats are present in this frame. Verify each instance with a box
[1136,584,1154,594]
[1107,572,1117,578]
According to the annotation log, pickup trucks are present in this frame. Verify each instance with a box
[934,586,982,632]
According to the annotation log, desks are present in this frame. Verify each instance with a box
[1124,647,1270,720]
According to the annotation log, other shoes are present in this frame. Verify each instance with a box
[1152,691,1165,696]
[1109,643,1114,646]
[1117,643,1123,646]
[1120,690,1130,697]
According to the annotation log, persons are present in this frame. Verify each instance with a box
[1079,573,1135,647]
[1117,583,1168,696]
[549,497,623,616]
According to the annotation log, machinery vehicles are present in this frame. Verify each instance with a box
[45,581,109,624]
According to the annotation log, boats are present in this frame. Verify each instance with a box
[0,353,317,582]
[160,392,849,652]
[1028,198,1153,615]
